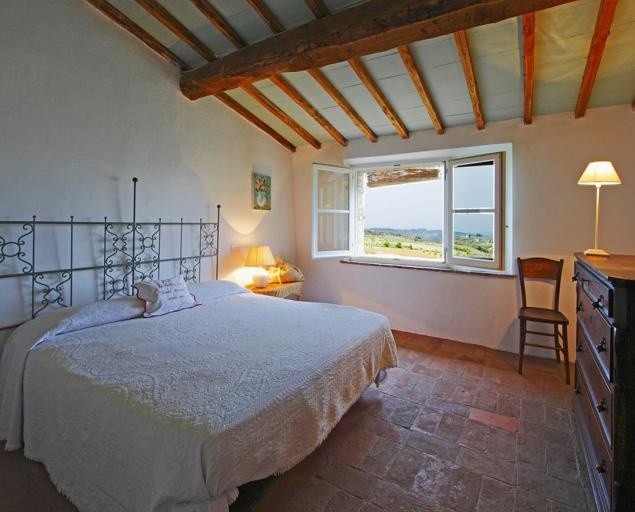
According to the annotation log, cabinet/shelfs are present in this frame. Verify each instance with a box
[575,253,635,512]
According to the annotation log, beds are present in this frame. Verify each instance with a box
[0,176,399,511]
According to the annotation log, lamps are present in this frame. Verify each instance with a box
[243,245,278,289]
[577,161,623,256]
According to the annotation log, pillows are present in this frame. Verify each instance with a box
[128,273,204,318]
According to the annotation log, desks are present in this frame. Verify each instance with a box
[244,281,304,302]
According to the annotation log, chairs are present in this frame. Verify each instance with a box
[515,255,571,384]
